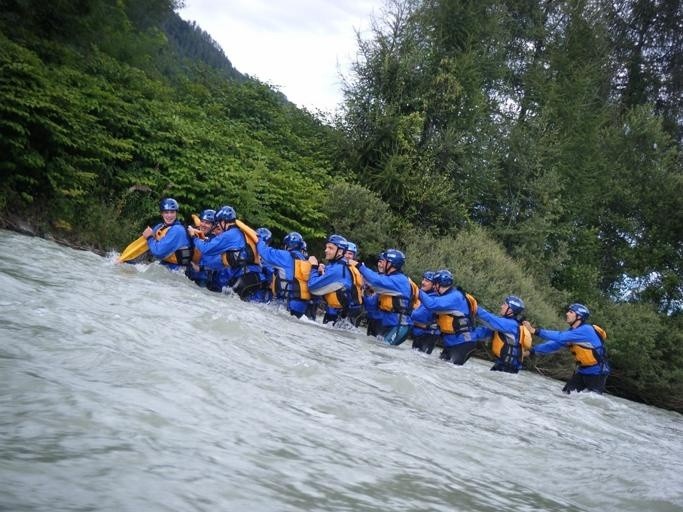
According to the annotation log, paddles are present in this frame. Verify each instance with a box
[118,236,150,260]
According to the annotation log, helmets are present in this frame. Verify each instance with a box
[434,270,454,286]
[505,296,525,314]
[257,228,272,246]
[570,303,589,319]
[328,234,357,259]
[160,198,179,211]
[199,209,216,222]
[423,272,435,283]
[378,249,405,268]
[283,232,307,252]
[216,206,236,221]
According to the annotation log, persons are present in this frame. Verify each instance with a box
[521,302,609,399]
[308,235,421,346]
[409,269,532,373]
[142,198,317,320]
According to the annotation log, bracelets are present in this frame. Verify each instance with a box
[533,328,540,335]
